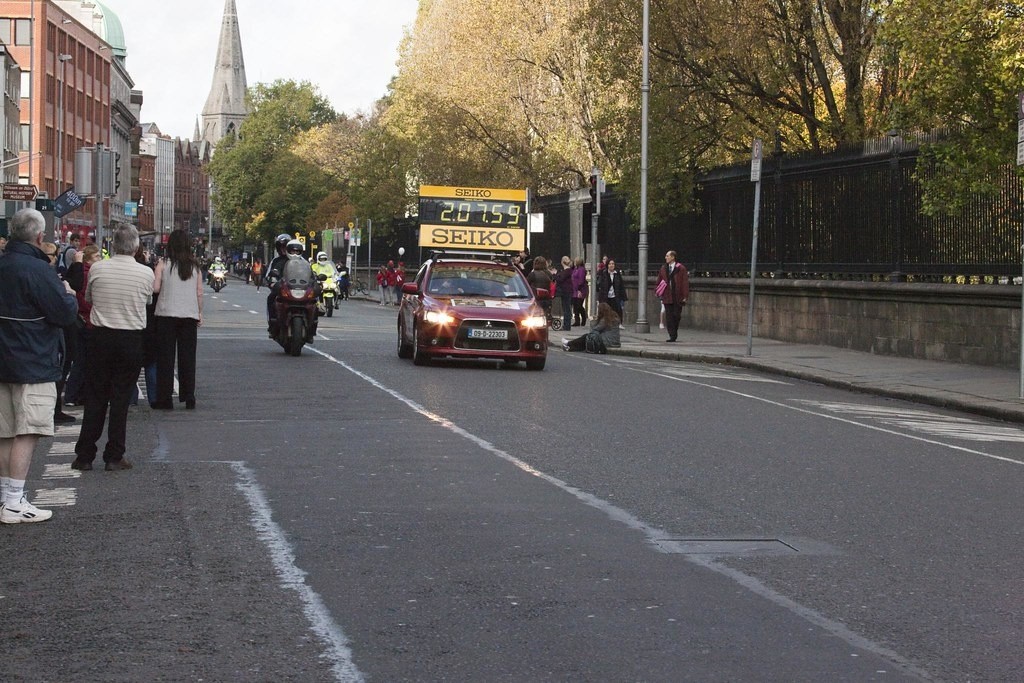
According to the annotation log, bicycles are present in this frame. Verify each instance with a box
[350,277,370,296]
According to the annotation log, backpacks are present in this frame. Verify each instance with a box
[57,246,77,275]
[585,330,607,354]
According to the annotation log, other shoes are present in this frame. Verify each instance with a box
[71,455,93,470]
[104,457,134,471]
[666,338,677,342]
[306,335,313,344]
[561,337,570,352]
[619,324,626,330]
[185,397,197,409]
[150,400,174,410]
[659,323,665,330]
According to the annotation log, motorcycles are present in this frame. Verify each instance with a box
[266,268,327,356]
[313,270,347,317]
[209,269,229,291]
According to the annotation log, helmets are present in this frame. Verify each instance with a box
[275,233,291,258]
[285,240,303,260]
[317,252,328,266]
[215,257,222,265]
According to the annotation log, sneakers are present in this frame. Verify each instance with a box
[0,493,53,524]
[53,412,76,423]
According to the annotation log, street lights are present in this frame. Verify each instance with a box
[208,182,215,255]
[58,52,77,196]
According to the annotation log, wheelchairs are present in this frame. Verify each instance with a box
[534,289,563,331]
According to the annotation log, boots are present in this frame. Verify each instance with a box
[581,315,587,326]
[572,317,581,326]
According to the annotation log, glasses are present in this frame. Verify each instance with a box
[45,251,58,257]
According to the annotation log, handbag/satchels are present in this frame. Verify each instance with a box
[655,279,667,296]
[382,278,388,288]
[549,281,556,299]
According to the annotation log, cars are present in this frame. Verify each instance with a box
[398,249,548,371]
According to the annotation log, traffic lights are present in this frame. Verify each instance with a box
[589,175,598,215]
[115,152,121,194]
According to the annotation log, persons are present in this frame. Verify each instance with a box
[654,251,689,342]
[222,256,250,278]
[41,224,209,471]
[375,260,405,306]
[0,209,80,523]
[511,247,628,331]
[308,252,349,309]
[265,234,324,344]
[207,257,227,286]
[252,261,264,293]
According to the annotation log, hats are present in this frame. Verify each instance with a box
[388,260,394,267]
[397,262,405,267]
